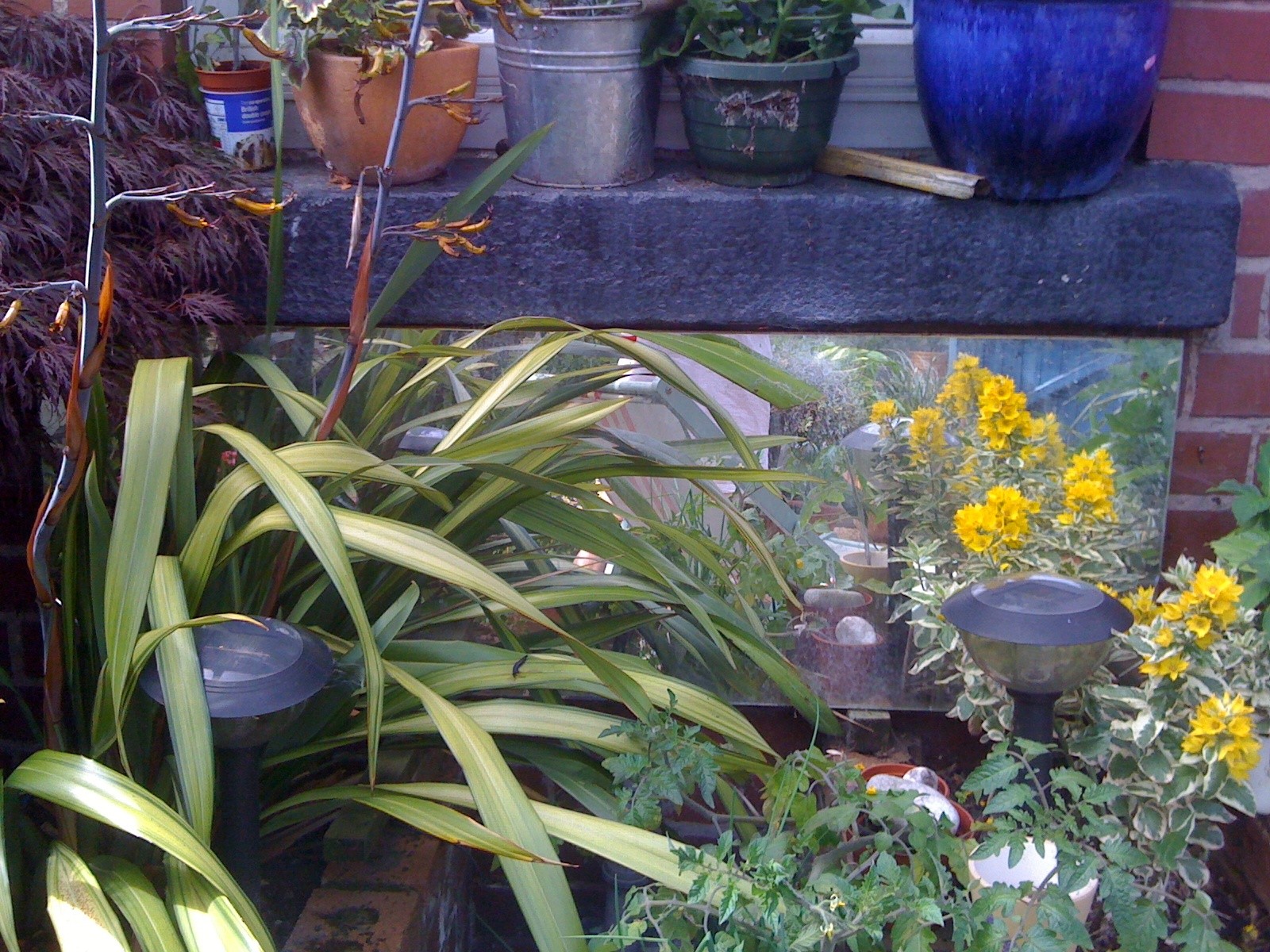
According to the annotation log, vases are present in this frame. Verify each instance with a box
[846,763,1100,952]
[908,1,1178,200]
[1237,713,1270,914]
[768,483,928,704]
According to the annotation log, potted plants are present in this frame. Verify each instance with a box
[264,0,493,186]
[655,0,888,189]
[633,452,844,700]
[167,5,281,170]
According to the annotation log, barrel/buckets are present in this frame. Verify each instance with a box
[761,585,885,703]
[967,836,1099,951]
[842,763,975,935]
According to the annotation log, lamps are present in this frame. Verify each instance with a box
[940,575,1135,702]
[397,426,447,456]
[838,412,961,495]
[125,621,330,756]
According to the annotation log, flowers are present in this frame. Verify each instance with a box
[866,358,1120,576]
[1089,550,1270,863]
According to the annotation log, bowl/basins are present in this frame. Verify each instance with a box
[838,552,890,585]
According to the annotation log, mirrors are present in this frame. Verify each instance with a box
[104,321,1180,710]
